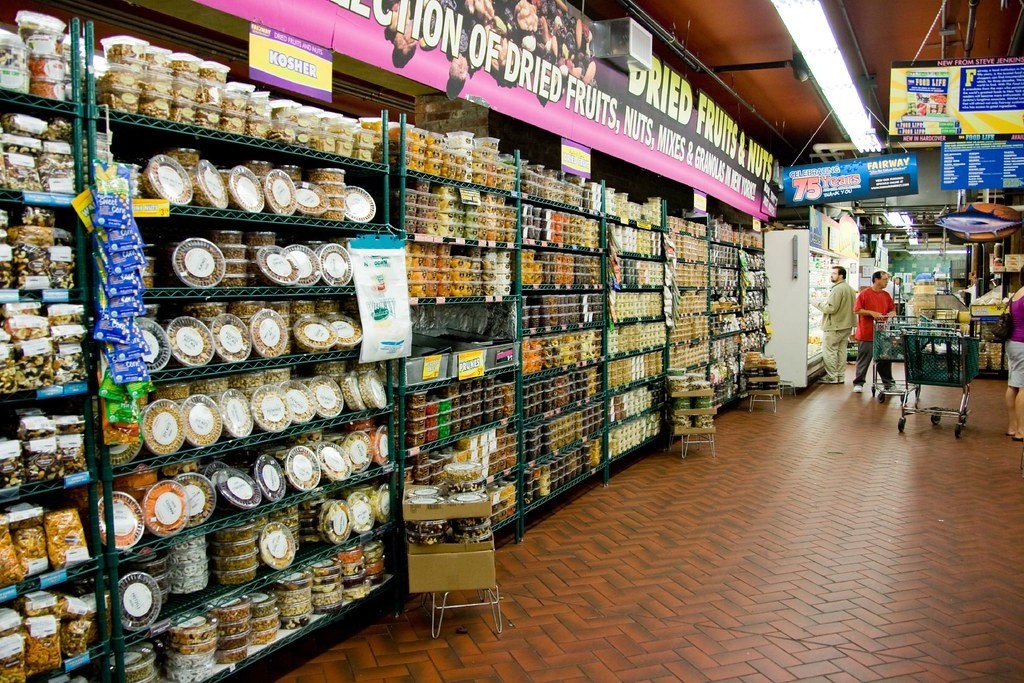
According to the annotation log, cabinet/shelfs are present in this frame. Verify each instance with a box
[808,253,849,377]
[0,17,764,682]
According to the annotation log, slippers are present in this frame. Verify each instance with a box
[1006,432,1015,436]
[1013,436,1023,441]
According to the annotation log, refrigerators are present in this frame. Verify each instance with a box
[764,229,859,387]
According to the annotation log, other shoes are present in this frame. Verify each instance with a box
[817,374,844,383]
[853,385,863,393]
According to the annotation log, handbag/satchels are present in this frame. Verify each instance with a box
[991,295,1014,339]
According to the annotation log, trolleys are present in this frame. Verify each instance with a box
[872,315,921,404]
[897,307,979,439]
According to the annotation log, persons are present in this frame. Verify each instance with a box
[853,271,903,393]
[811,266,857,384]
[887,273,904,312]
[1004,265,1024,442]
